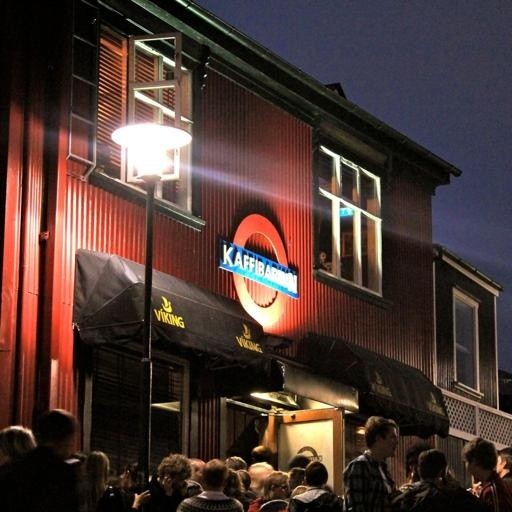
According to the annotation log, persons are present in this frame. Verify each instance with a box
[319,249,332,271]
[0,410,511,512]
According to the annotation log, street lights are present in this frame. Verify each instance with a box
[108,120,194,489]
